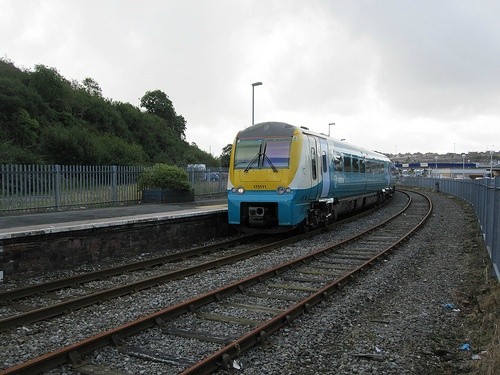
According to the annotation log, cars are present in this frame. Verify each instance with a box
[200,171,228,182]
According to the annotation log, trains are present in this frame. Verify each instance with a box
[227,121,400,236]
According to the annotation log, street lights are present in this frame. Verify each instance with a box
[462,153,467,170]
[489,150,495,180]
[251,82,263,126]
[434,156,439,171]
[328,122,336,137]
